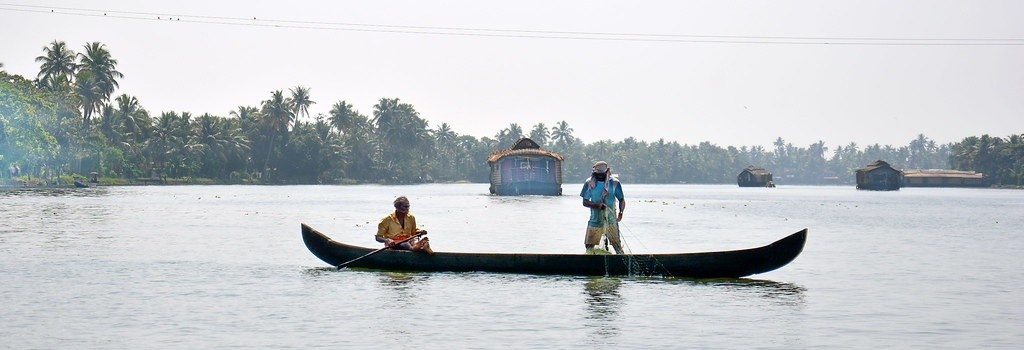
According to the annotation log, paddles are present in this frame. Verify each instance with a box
[336,232,422,270]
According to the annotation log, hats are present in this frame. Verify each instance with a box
[593,161,607,173]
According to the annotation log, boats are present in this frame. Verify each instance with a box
[855,159,904,191]
[300,222,807,279]
[736,165,773,187]
[487,138,565,195]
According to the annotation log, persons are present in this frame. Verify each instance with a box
[375,197,429,250]
[580,161,625,255]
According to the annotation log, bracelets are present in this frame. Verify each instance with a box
[619,211,623,214]
